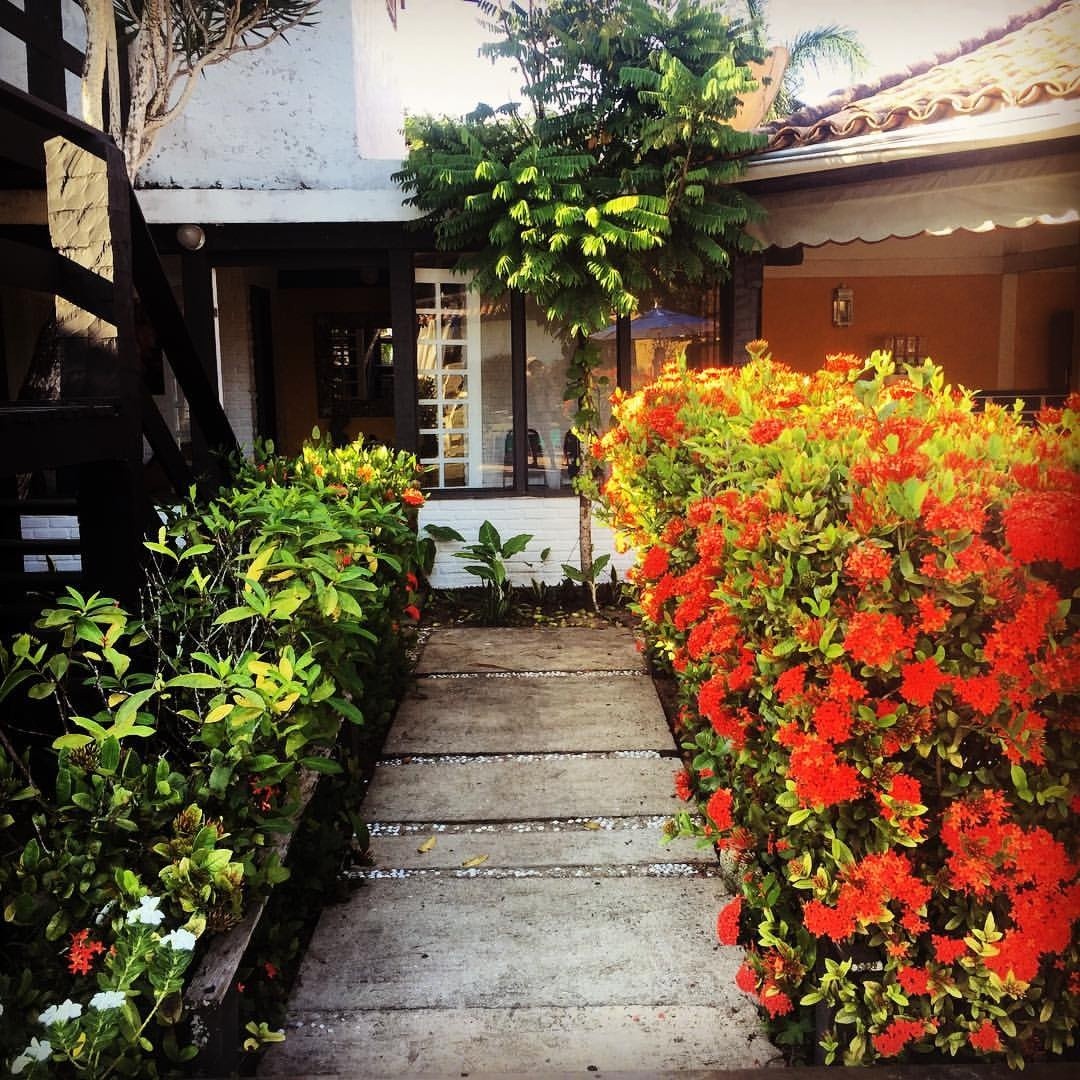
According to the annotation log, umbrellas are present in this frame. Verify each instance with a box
[587,307,720,346]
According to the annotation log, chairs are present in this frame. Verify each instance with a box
[559,429,605,489]
[502,428,546,488]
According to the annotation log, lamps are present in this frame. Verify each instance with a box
[832,282,855,328]
[178,223,205,250]
